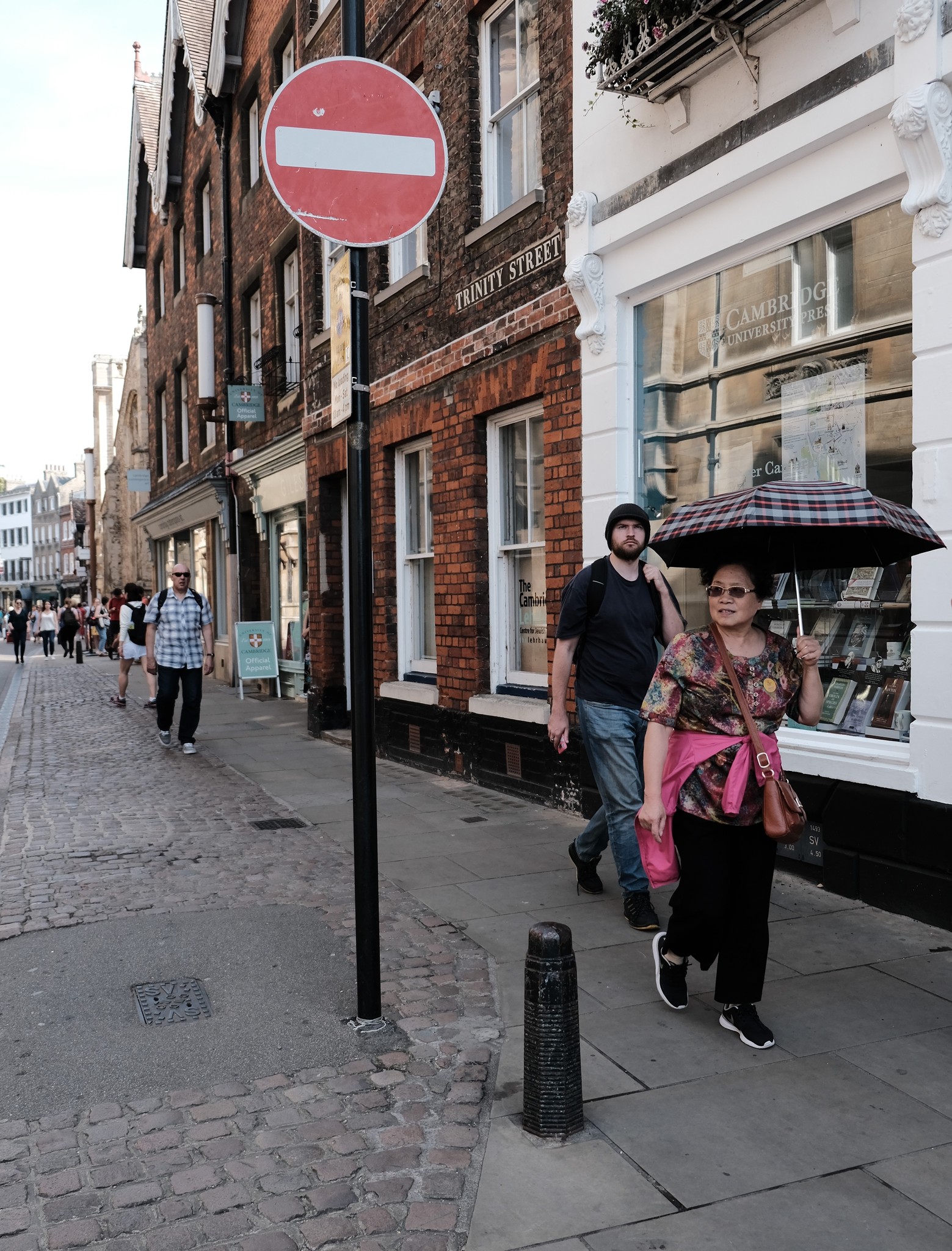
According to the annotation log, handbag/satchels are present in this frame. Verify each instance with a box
[102,616,110,626]
[31,617,36,622]
[86,616,99,626]
[761,769,807,846]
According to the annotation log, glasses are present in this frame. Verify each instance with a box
[171,572,191,578]
[705,585,758,599]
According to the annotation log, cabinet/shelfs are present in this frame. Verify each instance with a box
[706,554,917,759]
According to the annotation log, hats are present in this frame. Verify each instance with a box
[111,588,121,596]
[605,503,651,553]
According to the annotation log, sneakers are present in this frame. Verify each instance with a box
[182,743,197,754]
[652,931,693,1009]
[568,837,604,896]
[144,698,157,708]
[158,728,172,746]
[719,1002,775,1050]
[110,695,126,707]
[623,889,662,930]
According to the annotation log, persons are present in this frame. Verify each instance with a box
[58,597,80,659]
[144,563,215,754]
[8,599,30,663]
[547,502,687,930]
[0,585,148,660]
[37,600,58,660]
[111,582,157,707]
[301,580,309,641]
[636,551,823,1049]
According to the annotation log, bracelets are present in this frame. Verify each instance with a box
[117,646,123,648]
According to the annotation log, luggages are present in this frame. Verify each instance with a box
[6,631,14,644]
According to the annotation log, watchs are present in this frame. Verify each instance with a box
[206,653,214,657]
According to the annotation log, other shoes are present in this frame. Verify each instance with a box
[92,649,120,660]
[21,657,24,663]
[50,654,55,660]
[135,657,141,665]
[70,655,74,658]
[64,649,69,657]
[44,656,49,661]
[16,658,19,664]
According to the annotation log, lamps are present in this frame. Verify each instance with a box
[190,292,240,428]
[82,447,98,503]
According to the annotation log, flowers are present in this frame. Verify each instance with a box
[573,0,716,79]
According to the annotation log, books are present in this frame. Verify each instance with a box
[762,567,911,728]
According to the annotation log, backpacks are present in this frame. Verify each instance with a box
[62,606,74,624]
[122,602,147,646]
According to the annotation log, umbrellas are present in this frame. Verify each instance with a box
[651,481,946,631]
